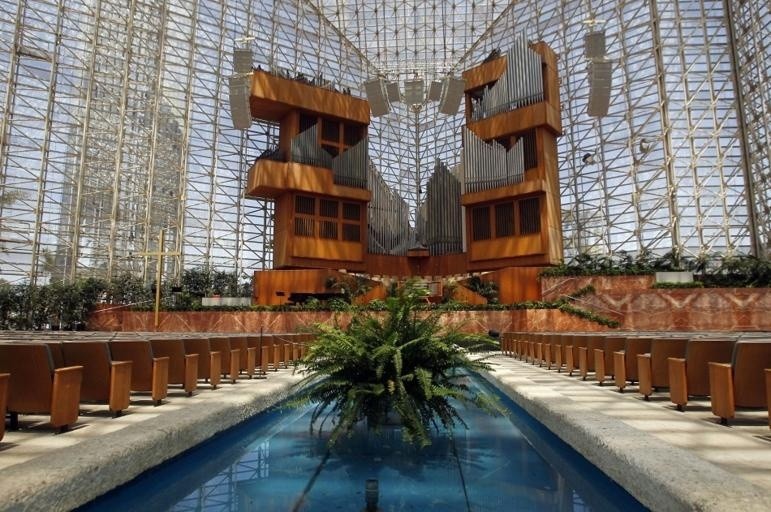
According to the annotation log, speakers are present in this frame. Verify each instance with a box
[363,77,465,118]
[229,75,253,129]
[653,271,693,283]
[585,30,607,57]
[584,62,612,116]
[234,49,253,73]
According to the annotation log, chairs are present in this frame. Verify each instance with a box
[49,330,318,415]
[3,341,85,433]
[499,329,771,441]
[1,372,10,441]
